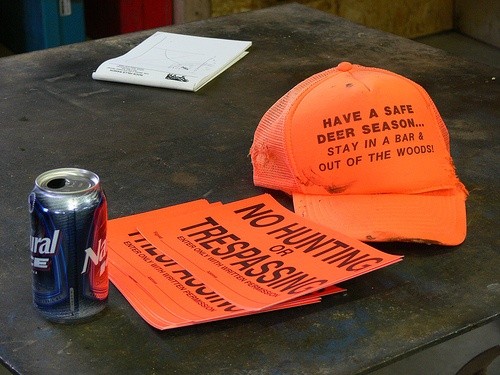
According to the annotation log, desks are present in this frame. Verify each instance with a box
[0,2,500,375]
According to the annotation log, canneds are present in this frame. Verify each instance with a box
[26,166,110,325]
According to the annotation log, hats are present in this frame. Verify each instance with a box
[252,61,470,247]
